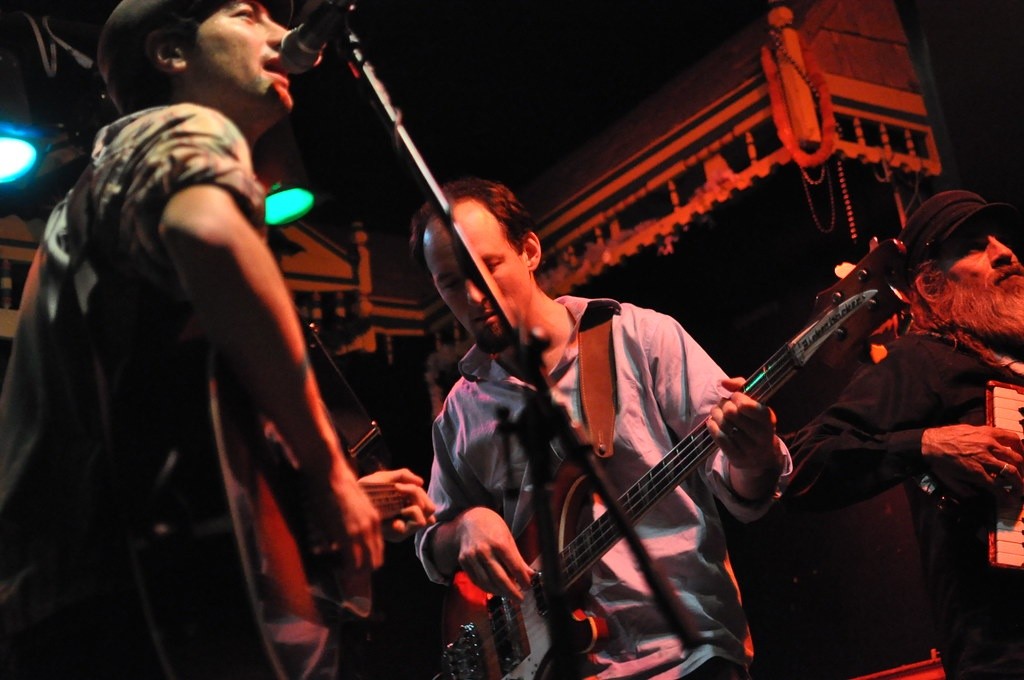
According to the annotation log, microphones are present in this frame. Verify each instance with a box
[277,0,354,74]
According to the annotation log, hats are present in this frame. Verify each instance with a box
[895,190,1017,285]
[97,0,229,115]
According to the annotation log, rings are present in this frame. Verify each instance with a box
[1001,462,1009,475]
[729,427,739,435]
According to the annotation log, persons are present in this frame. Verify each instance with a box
[0,0,439,680]
[786,188,1024,680]
[405,177,792,680]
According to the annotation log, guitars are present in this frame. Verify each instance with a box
[111,334,423,679]
[440,238,911,679]
